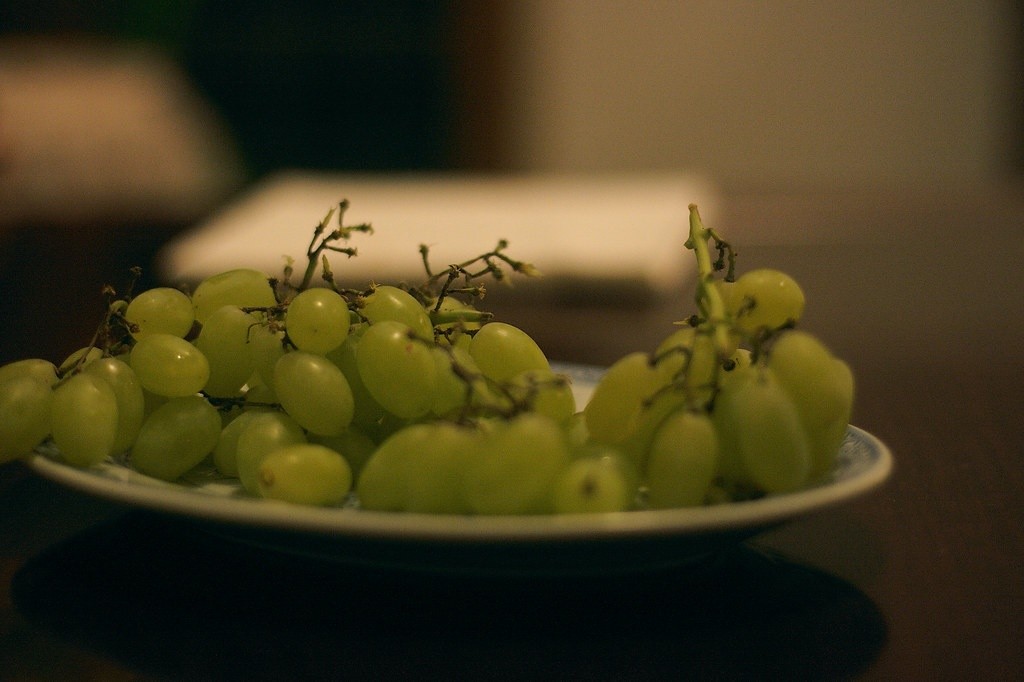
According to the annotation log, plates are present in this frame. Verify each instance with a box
[18,361,895,541]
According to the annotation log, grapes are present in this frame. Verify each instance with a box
[1,271,855,519]
